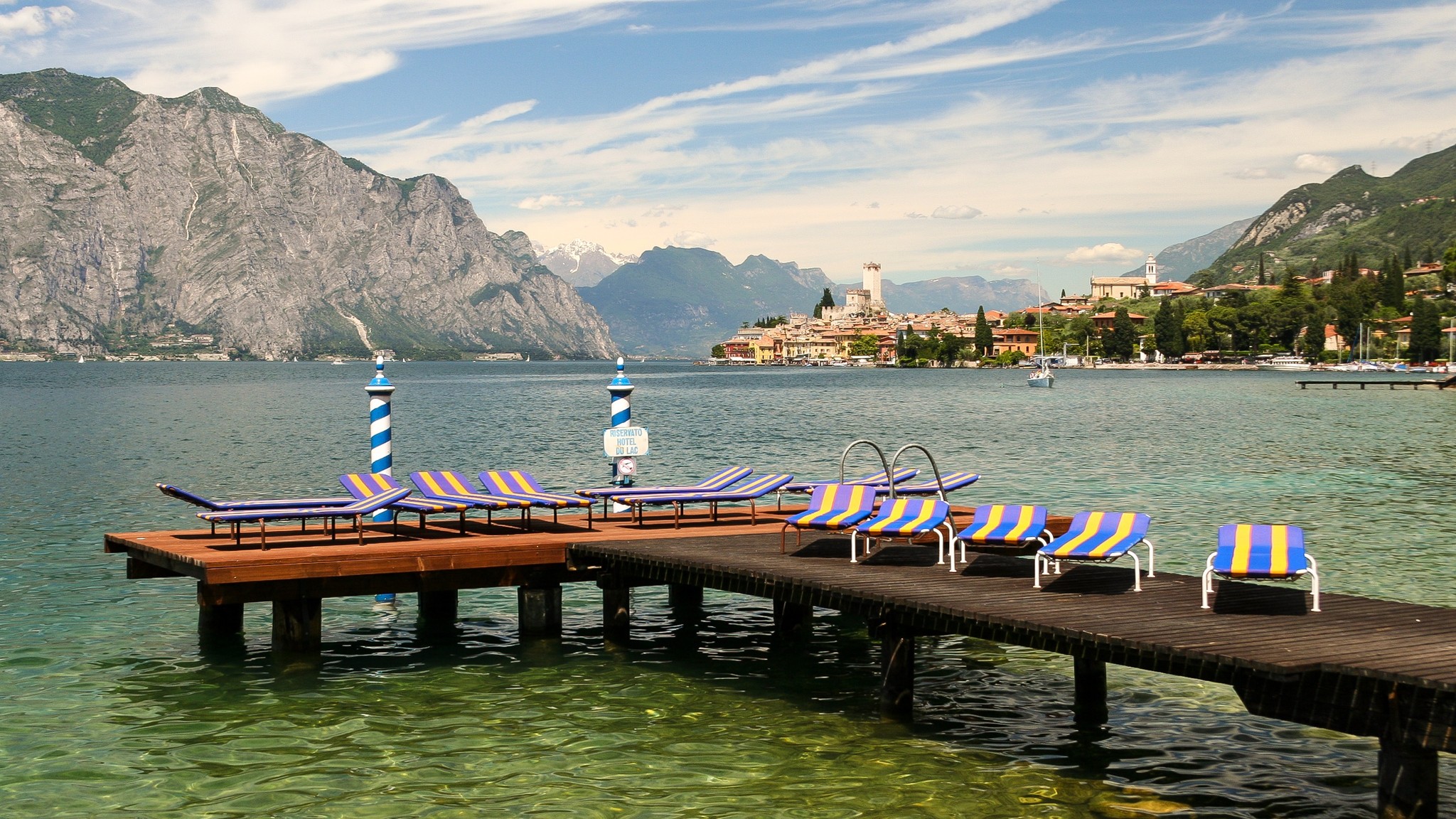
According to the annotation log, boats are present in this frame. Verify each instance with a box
[1255,358,1312,372]
[831,361,877,367]
[282,356,290,363]
[1324,361,1456,373]
[330,359,350,365]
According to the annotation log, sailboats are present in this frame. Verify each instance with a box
[1026,257,1054,388]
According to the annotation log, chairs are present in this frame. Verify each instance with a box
[156,461,1156,598]
[1202,525,1325,614]
